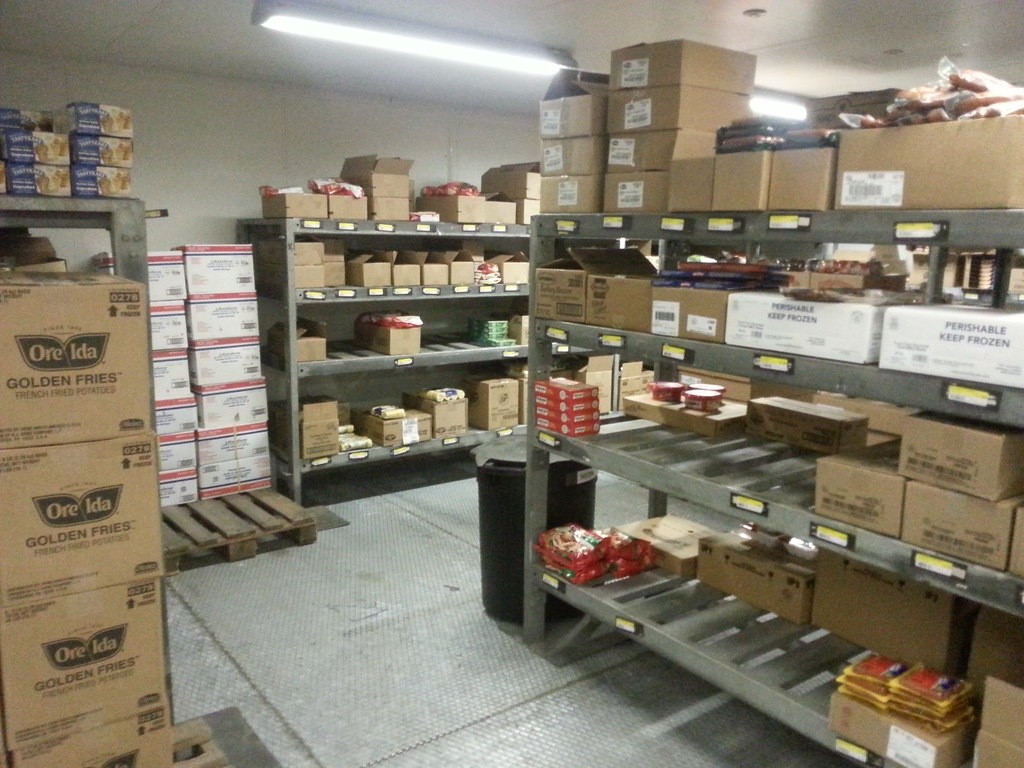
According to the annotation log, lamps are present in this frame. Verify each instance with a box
[746,86,812,118]
[250,1,578,76]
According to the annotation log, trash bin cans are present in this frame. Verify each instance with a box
[469,434,598,624]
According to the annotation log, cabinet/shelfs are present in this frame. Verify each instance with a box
[522,208,1023,768]
[236,216,530,508]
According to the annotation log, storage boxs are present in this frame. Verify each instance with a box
[0,39,1024,767]
